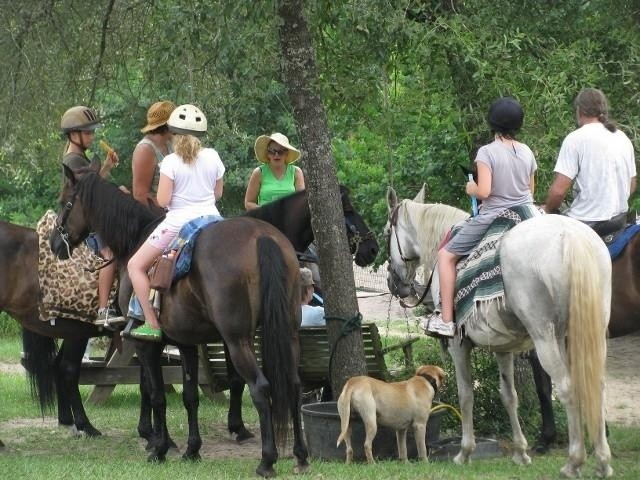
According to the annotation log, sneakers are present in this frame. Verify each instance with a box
[420,313,457,339]
[129,319,163,343]
[94,307,127,326]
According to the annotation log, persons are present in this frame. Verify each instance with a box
[543,87,638,237]
[297,267,326,330]
[60,105,130,330]
[418,96,538,338]
[131,100,176,209]
[127,103,226,339]
[244,132,322,293]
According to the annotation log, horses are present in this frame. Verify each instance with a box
[21,184,380,446]
[0,220,183,456]
[48,151,311,478]
[456,162,640,457]
[383,182,614,480]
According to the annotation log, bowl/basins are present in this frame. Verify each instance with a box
[299,400,448,462]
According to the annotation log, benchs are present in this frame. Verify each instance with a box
[198,325,422,401]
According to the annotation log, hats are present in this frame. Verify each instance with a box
[254,132,301,166]
[141,100,178,135]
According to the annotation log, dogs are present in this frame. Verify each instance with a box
[336,364,448,466]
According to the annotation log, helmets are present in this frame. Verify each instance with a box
[166,103,208,137]
[60,105,104,134]
[486,96,526,133]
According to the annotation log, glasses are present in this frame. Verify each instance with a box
[265,147,288,156]
[311,282,318,289]
